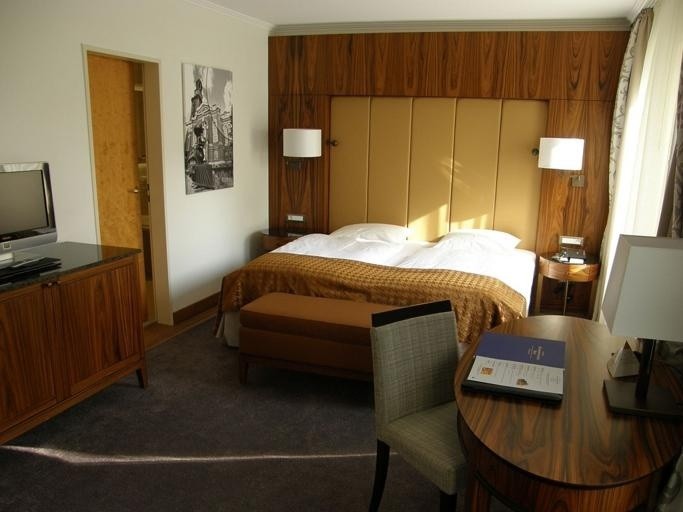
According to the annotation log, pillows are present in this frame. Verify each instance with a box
[327,222,416,242]
[434,228,521,249]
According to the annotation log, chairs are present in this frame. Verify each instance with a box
[368,296,465,510]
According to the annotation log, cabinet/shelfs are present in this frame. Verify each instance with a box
[0,240,153,446]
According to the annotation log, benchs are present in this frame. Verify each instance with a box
[238,291,400,388]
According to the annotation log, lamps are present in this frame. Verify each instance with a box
[597,231,682,421]
[538,136,587,188]
[280,127,322,170]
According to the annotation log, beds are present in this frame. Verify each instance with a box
[215,224,538,366]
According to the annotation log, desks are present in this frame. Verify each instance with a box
[533,250,598,317]
[259,226,313,257]
[450,307,682,512]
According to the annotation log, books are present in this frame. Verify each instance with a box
[460,332,566,406]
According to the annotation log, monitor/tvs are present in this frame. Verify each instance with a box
[0,162,57,269]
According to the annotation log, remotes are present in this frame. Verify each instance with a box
[11,255,44,268]
